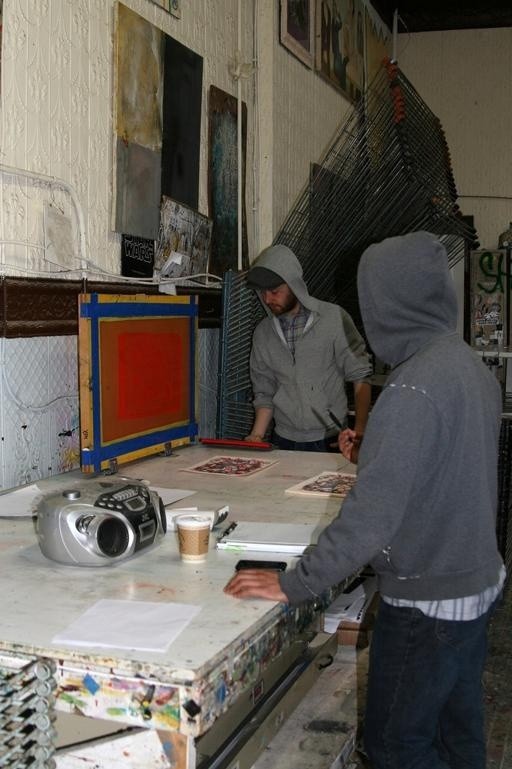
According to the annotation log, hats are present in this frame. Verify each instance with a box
[245,267,283,290]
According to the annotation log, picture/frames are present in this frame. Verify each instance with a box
[279,0,367,108]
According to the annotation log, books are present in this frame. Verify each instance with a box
[284,468,357,499]
[52,596,202,653]
[147,484,199,508]
[217,518,325,556]
[153,728,197,769]
[1,484,46,517]
[163,501,229,532]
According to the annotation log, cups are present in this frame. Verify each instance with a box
[175,514,212,564]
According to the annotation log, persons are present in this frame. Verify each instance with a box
[224,232,509,769]
[238,243,375,455]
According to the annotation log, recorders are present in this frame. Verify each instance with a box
[35,474,169,566]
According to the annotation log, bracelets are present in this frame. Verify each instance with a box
[250,432,264,440]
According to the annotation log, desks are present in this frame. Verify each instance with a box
[0,443,390,769]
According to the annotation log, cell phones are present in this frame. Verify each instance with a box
[235,558,287,572]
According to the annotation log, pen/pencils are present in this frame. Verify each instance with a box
[328,410,356,444]
